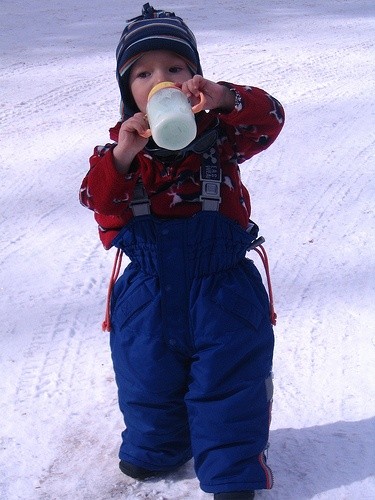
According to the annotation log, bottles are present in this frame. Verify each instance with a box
[138,81,207,151]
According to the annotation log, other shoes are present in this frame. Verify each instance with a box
[119,460,162,480]
[214,490,255,500]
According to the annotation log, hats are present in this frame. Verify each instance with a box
[115,2,203,122]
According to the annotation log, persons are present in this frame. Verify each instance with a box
[79,1,284,500]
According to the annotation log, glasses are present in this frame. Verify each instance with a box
[143,118,219,166]
